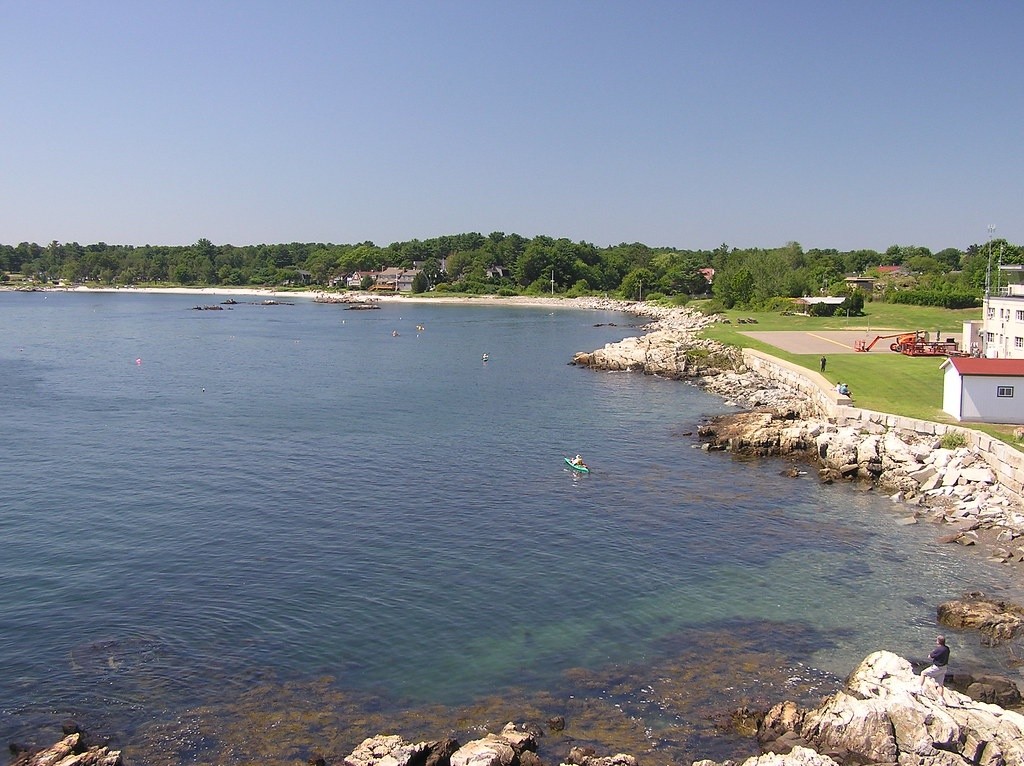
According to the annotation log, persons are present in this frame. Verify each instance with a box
[836,381,853,398]
[820,355,827,373]
[935,330,942,345]
[923,330,931,345]
[910,635,950,706]
[572,454,584,466]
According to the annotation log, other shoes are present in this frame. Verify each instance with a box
[915,689,922,694]
[940,700,946,705]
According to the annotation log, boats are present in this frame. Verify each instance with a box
[483,355,488,361]
[564,458,590,473]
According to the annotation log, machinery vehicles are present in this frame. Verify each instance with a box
[866,331,930,351]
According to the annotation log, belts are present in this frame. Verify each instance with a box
[935,664,945,666]
